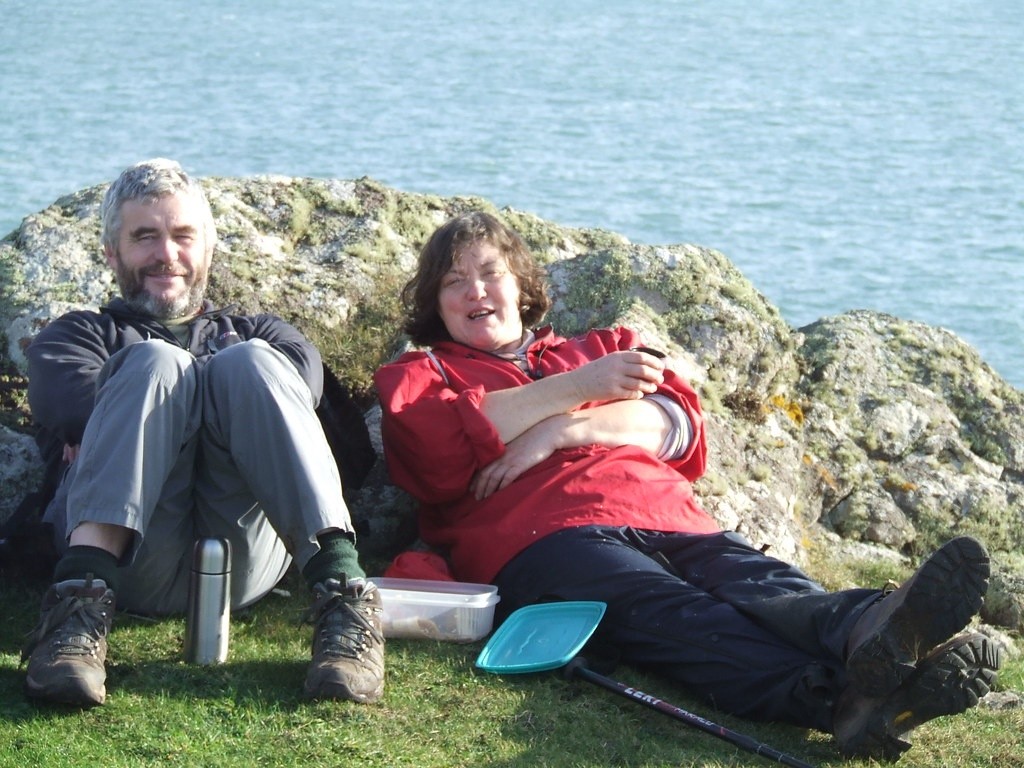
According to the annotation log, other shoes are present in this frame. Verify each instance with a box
[830,632,1002,766]
[842,533,993,701]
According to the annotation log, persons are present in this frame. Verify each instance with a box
[373,211,1000,759]
[18,157,386,707]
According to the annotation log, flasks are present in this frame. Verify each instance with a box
[186,536,232,667]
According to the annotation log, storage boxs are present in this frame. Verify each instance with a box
[365,577,501,643]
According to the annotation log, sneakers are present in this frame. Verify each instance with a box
[298,572,387,702]
[16,571,107,706]
